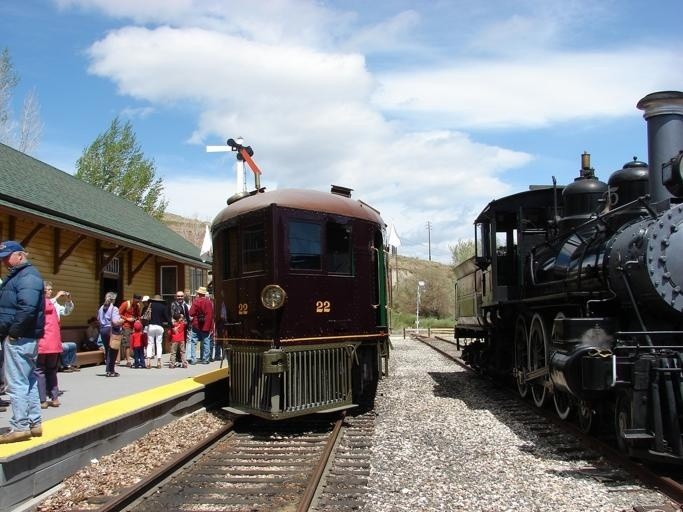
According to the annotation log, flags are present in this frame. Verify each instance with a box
[388,223,402,248]
[200,225,213,263]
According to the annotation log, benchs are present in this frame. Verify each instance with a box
[76,350,104,369]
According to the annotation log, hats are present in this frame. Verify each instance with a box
[105,292,118,300]
[1,240,23,257]
[134,293,164,302]
[134,320,143,330]
[195,287,209,295]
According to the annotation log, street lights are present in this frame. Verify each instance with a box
[415,280,425,331]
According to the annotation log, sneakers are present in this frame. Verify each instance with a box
[125,359,188,367]
[106,372,120,377]
[0,423,42,443]
[0,390,63,409]
[64,365,80,372]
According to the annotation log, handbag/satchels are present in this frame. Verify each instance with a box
[198,314,205,325]
[143,308,152,320]
[109,334,122,350]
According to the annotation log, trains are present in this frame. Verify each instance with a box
[451,91,682,465]
[209,138,393,421]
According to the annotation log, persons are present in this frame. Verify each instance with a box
[86,287,227,377]
[0,240,80,443]
